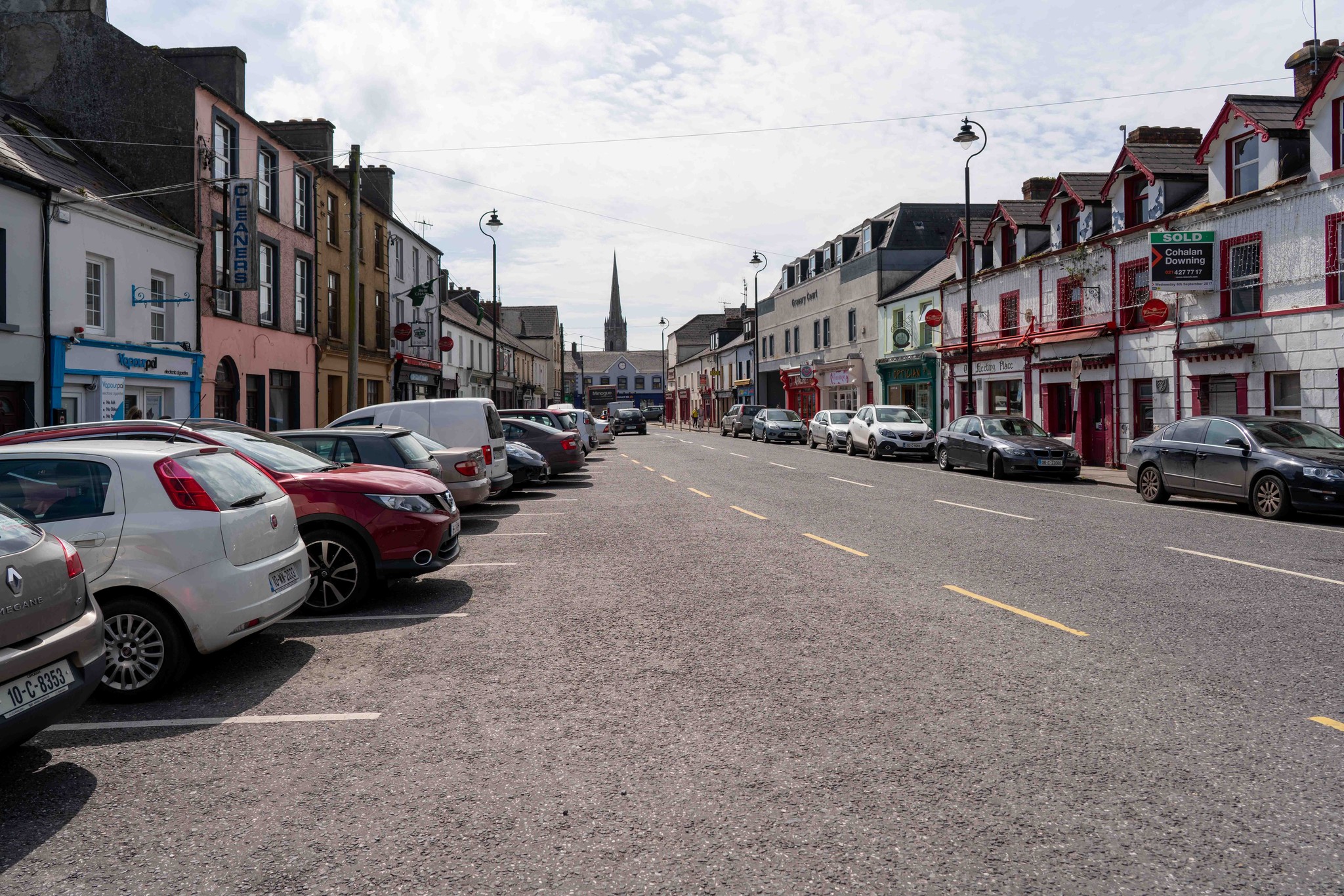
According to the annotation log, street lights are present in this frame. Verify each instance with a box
[749,250,767,406]
[659,316,669,425]
[953,116,987,417]
[580,335,585,409]
[479,207,504,408]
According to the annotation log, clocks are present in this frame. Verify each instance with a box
[618,362,626,369]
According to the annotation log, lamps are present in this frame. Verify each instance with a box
[146,341,193,352]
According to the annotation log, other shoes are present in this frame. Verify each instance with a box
[693,425,694,428]
[698,425,699,427]
[696,427,698,428]
[701,426,703,427]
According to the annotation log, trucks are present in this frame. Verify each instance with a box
[607,401,636,423]
[583,385,617,419]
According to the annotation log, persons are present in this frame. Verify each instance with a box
[907,405,914,409]
[158,416,171,420]
[692,404,704,428]
[125,405,142,420]
[776,404,781,409]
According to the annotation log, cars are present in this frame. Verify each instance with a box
[0,502,107,757]
[640,406,666,422]
[751,408,808,445]
[487,404,646,499]
[935,414,1081,480]
[808,409,858,452]
[0,440,312,707]
[267,424,491,505]
[1125,415,1344,521]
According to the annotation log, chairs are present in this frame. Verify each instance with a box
[43,462,96,520]
[0,475,37,520]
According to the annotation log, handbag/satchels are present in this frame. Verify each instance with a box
[691,413,694,418]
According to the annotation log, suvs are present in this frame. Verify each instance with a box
[846,404,937,461]
[0,417,461,617]
[721,404,768,438]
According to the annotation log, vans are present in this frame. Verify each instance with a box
[324,397,513,492]
